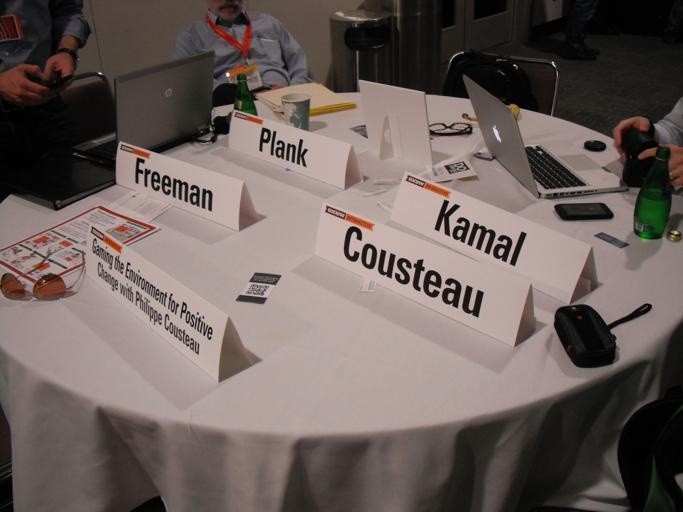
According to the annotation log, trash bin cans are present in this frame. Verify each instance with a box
[329,9,399,93]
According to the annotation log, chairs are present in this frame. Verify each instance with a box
[59,69,115,146]
[437,47,561,117]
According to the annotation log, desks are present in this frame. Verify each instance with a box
[0,75,683,511]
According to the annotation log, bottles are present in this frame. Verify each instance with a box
[632,146,672,241]
[232,72,258,117]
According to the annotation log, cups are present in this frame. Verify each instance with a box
[280,93,310,130]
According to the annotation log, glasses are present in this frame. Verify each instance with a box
[1,251,88,300]
[428,122,473,137]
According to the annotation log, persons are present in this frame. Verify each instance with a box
[613,96,683,187]
[173,0,312,133]
[617,385,681,512]
[0,0,92,197]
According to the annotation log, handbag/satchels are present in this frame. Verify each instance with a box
[444,52,538,113]
[555,304,618,366]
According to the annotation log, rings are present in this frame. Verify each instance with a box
[669,171,674,181]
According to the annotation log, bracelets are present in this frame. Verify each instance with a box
[54,48,79,70]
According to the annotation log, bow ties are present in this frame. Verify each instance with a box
[218,12,249,27]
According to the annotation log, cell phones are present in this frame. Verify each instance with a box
[552,201,616,222]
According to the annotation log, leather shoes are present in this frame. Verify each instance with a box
[564,41,600,60]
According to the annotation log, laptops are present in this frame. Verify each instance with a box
[460,72,630,200]
[73,49,217,168]
